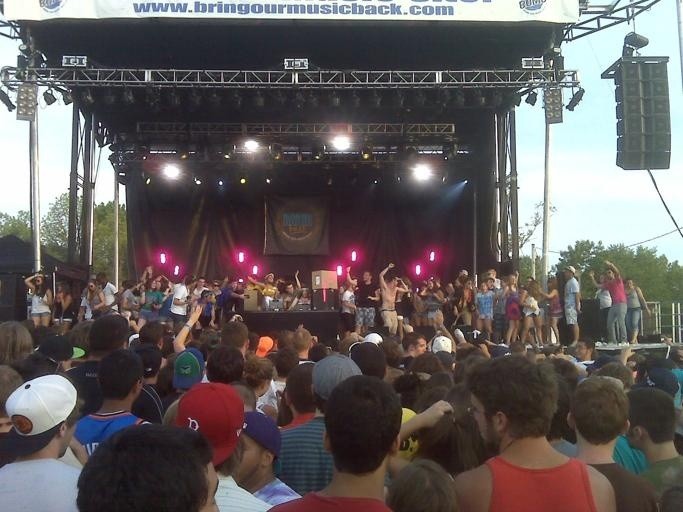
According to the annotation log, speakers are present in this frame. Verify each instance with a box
[613,62,672,171]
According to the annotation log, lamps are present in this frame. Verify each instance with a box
[545,47,585,112]
[41,87,538,109]
[622,9,649,49]
[176,141,459,162]
[0,44,31,112]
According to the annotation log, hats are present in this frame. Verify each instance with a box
[256,336,273,357]
[135,342,161,377]
[431,335,452,358]
[243,412,282,474]
[632,368,678,396]
[5,374,77,457]
[39,334,85,360]
[562,266,575,273]
[175,381,243,467]
[312,355,364,401]
[172,349,204,390]
[349,342,385,379]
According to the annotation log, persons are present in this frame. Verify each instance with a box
[454,355,616,508]
[567,376,662,508]
[273,353,362,496]
[2,373,82,509]
[625,387,682,508]
[178,383,275,509]
[270,375,407,507]
[0,261,683,475]
[386,459,457,508]
[76,424,220,508]
